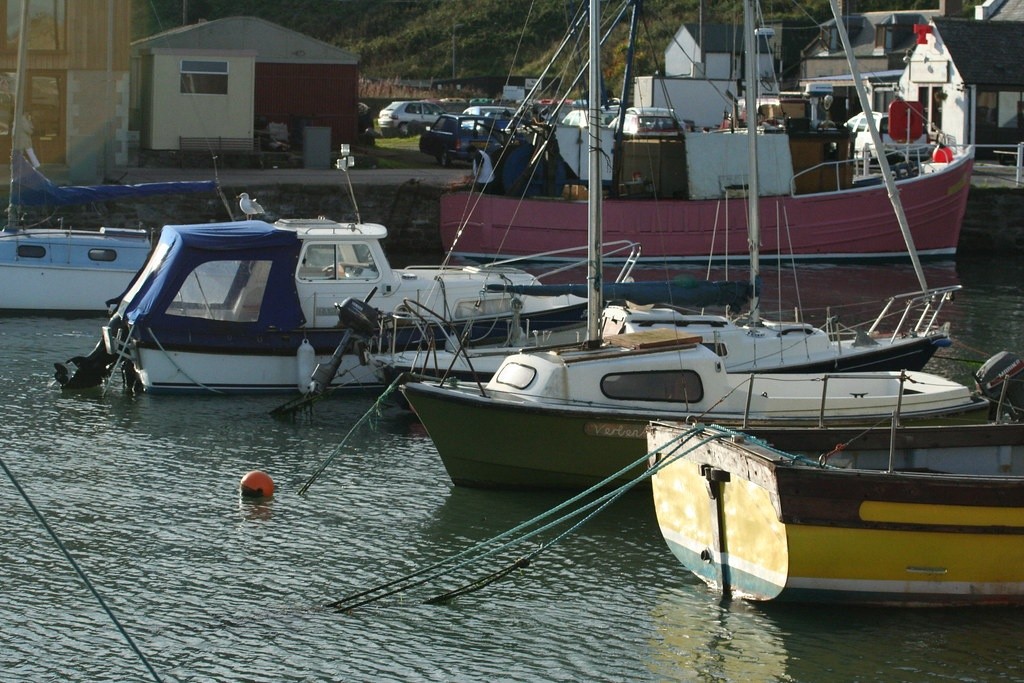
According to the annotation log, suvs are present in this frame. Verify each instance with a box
[838,110,930,161]
[720,96,785,129]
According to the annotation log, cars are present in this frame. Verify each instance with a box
[523,102,612,128]
[420,113,507,167]
[378,98,454,138]
[458,104,517,133]
[608,107,685,137]
[601,97,620,112]
[440,97,588,106]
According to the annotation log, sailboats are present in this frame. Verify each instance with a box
[340,3,1024,486]
[442,0,977,269]
[0,1,236,313]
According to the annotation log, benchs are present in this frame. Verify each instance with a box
[177,135,260,169]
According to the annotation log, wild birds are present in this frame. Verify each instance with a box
[236,193,265,221]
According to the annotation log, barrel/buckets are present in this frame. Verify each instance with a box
[302,126,333,170]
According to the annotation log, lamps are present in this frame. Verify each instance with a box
[923,50,942,63]
[903,49,923,63]
[337,144,362,223]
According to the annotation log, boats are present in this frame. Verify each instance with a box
[52,212,642,400]
[642,372,1023,612]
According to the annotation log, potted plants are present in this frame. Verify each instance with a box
[934,92,948,103]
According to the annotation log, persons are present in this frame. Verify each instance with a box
[465,145,496,195]
[12,105,45,171]
